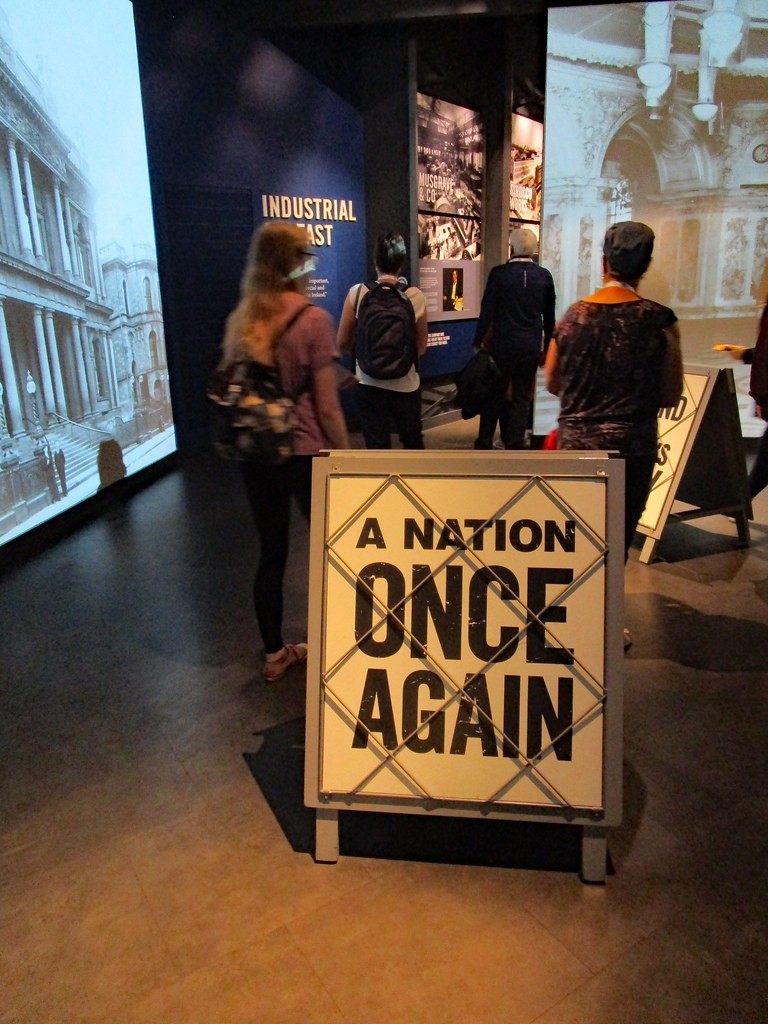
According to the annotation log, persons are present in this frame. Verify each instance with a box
[418,154,481,259]
[54,448,68,496]
[97,438,126,493]
[337,233,427,450]
[447,270,462,311]
[545,221,684,569]
[221,222,351,681]
[728,291,768,523]
[472,229,556,450]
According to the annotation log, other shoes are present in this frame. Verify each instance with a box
[623,628,633,650]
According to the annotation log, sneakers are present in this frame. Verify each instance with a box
[261,643,308,682]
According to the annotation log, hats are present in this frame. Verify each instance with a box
[603,221,656,273]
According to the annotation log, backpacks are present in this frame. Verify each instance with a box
[203,303,316,466]
[354,280,417,381]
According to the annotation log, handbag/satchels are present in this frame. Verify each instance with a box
[453,349,492,420]
[543,428,559,450]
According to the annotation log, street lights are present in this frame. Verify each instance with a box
[131,375,141,417]
[25,369,47,455]
[139,375,147,414]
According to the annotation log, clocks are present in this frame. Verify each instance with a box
[752,143,768,163]
